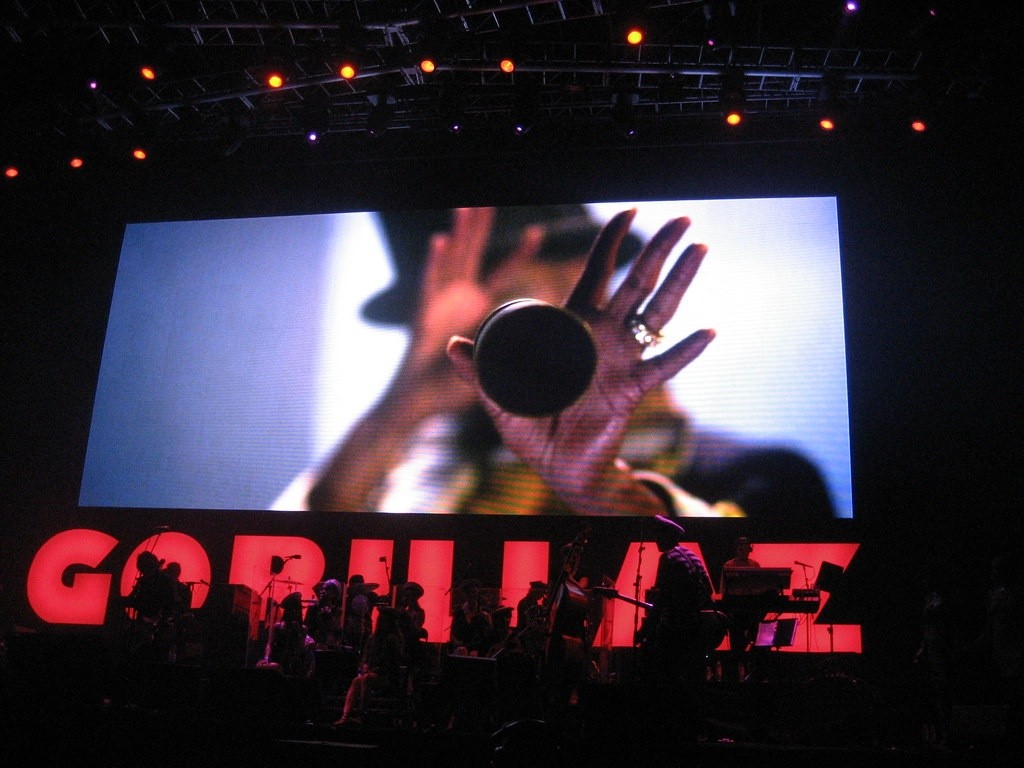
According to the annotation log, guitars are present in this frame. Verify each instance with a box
[591,581,730,661]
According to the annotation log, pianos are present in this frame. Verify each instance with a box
[700,593,825,613]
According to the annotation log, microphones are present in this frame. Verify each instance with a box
[153,525,170,531]
[285,554,301,559]
[379,556,387,562]
[794,561,812,568]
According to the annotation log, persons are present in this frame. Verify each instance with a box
[911,555,1023,750]
[634,515,713,646]
[719,537,761,650]
[518,581,550,624]
[256,575,425,725]
[167,563,191,608]
[299,201,832,518]
[448,580,489,729]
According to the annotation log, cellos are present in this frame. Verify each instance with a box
[539,520,595,670]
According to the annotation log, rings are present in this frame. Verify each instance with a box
[629,316,663,348]
